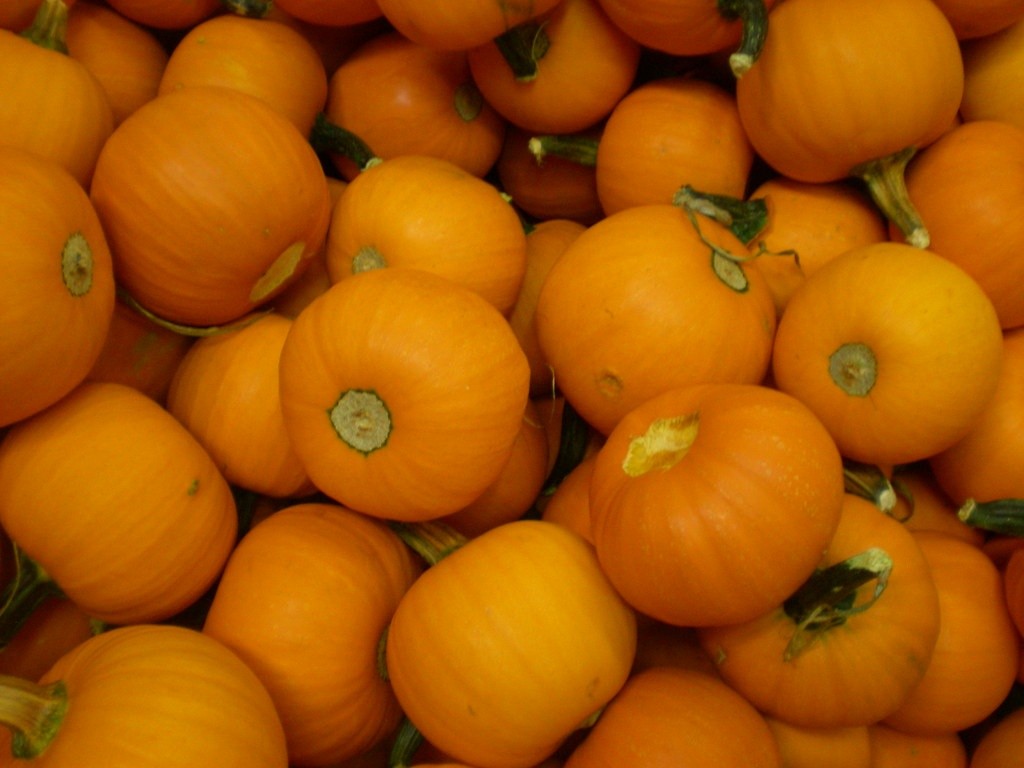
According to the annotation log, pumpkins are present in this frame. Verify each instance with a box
[0,0,1024,768]
